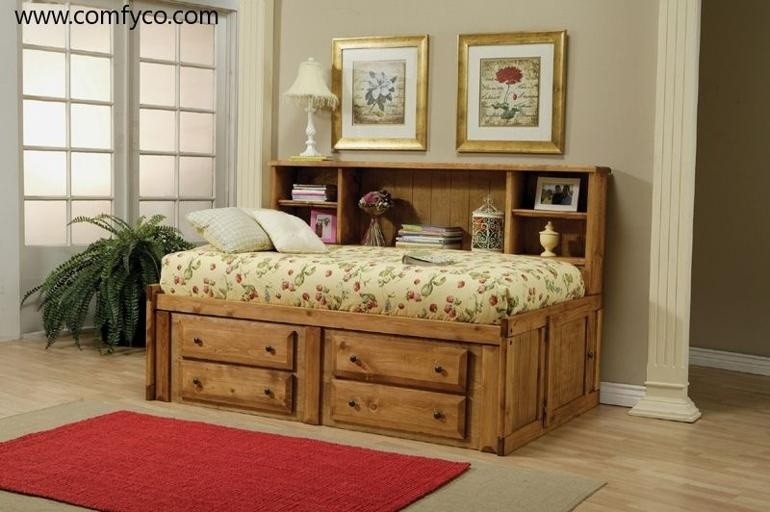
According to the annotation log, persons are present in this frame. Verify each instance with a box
[316,218,322,238]
[542,189,552,204]
[552,185,562,205]
[561,185,572,205]
[323,218,331,239]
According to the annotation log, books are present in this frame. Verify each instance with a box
[292,183,338,202]
[394,224,464,250]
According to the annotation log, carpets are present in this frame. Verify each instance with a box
[0,408,472,512]
[0,398,607,512]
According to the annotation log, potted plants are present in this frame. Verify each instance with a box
[21,212,191,356]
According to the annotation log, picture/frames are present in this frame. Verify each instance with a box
[310,207,337,245]
[455,29,568,155]
[534,175,582,213]
[330,34,431,152]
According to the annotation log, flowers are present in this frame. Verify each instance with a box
[358,190,395,247]
[493,66,525,120]
[363,71,397,112]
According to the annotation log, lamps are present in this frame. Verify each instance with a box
[283,58,340,157]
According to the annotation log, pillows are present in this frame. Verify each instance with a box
[242,206,330,255]
[186,207,274,254]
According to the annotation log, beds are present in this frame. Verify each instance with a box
[142,160,611,455]
